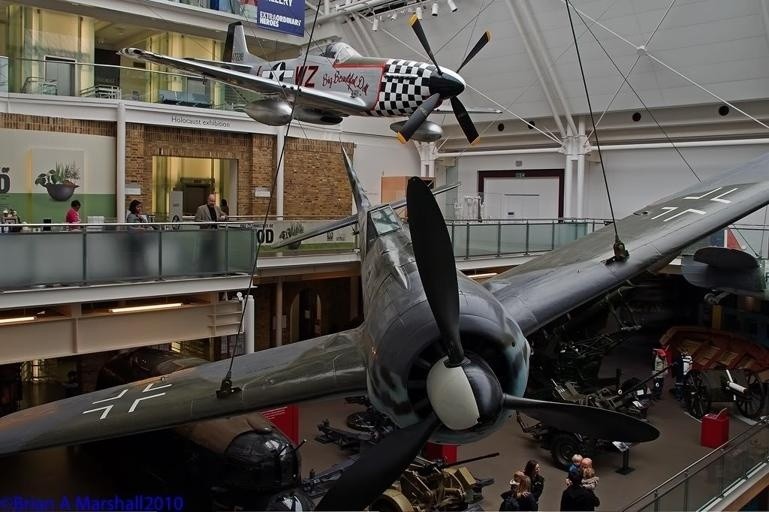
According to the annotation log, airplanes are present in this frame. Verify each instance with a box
[118,21,490,148]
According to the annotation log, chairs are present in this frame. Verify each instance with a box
[158,89,214,109]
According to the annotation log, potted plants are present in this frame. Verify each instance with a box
[34,160,80,201]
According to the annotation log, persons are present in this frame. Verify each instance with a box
[220,198,230,215]
[66,199,81,230]
[128,200,153,230]
[496,453,601,512]
[194,195,229,230]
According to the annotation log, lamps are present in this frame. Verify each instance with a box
[371,0,458,32]
